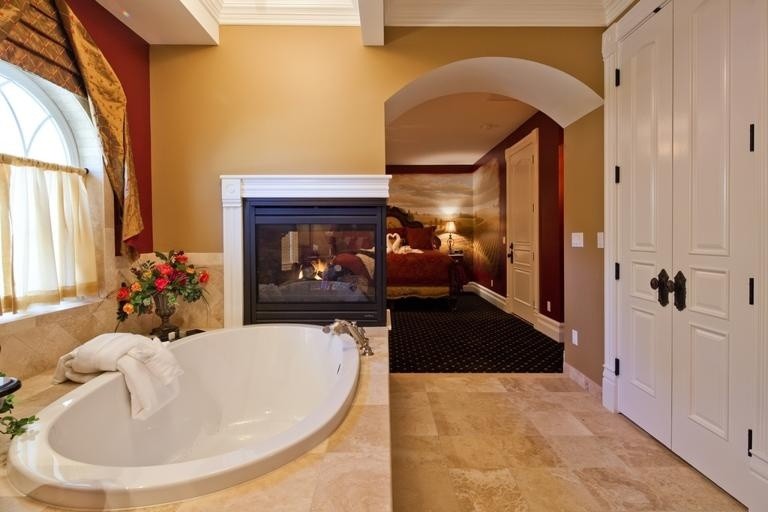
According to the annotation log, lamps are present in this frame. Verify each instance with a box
[445,222,457,255]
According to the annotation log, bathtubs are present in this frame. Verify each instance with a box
[8,323,361,510]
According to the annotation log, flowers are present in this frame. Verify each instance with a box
[92,250,212,335]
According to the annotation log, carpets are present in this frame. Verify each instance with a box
[390,291,565,372]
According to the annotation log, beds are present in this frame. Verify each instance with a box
[320,206,459,312]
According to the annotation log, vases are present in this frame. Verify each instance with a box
[150,293,179,342]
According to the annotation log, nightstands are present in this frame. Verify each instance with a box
[449,251,467,283]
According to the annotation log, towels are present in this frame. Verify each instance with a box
[53,330,182,419]
[393,233,424,254]
[360,233,393,253]
[258,283,283,302]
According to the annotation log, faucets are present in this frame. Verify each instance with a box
[332,321,374,356]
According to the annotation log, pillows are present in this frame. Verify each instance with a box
[405,227,435,249]
[384,227,406,245]
[326,230,372,250]
